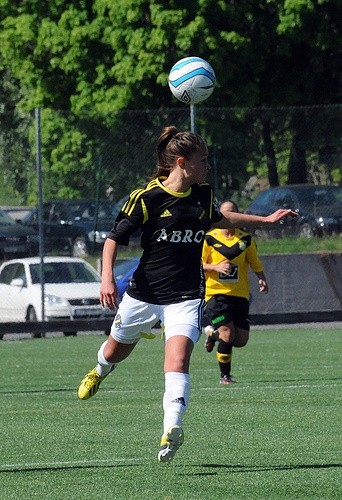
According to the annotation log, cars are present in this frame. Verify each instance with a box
[0,209,43,259]
[0,255,116,337]
[239,183,342,242]
[24,198,127,259]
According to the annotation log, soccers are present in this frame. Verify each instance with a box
[168,57,216,106]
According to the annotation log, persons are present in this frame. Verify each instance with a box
[200,259,213,351]
[200,199,269,386]
[77,127,300,465]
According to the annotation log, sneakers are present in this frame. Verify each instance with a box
[218,376,235,385]
[157,426,184,463]
[205,339,216,353]
[76,366,103,400]
